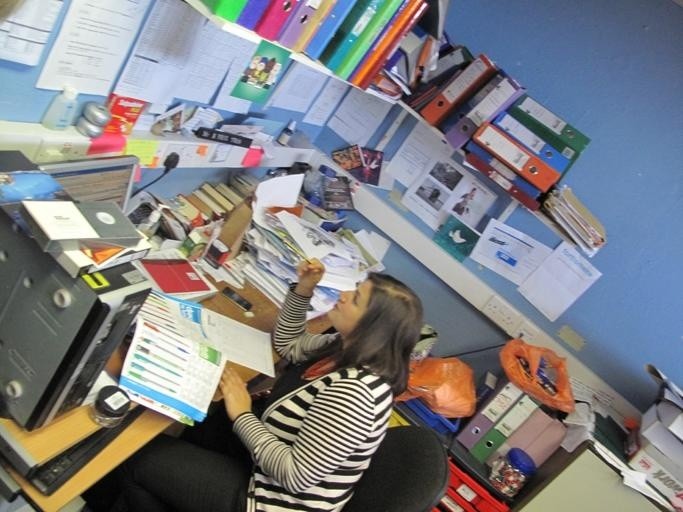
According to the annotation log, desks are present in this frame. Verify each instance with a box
[0,194,336,512]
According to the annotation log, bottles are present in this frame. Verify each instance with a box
[40,84,78,131]
[275,119,297,147]
[486,447,537,499]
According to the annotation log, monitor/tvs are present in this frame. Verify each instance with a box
[36,156,139,214]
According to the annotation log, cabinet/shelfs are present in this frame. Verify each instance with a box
[510,439,671,512]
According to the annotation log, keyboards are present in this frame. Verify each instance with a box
[29,405,147,497]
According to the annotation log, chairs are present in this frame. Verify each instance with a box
[341,425,449,512]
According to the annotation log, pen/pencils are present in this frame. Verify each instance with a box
[282,240,313,265]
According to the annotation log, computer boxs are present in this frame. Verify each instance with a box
[1,150,153,433]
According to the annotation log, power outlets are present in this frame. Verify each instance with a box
[483,296,540,344]
[162,144,188,168]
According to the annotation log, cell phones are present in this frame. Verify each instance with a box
[221,286,252,312]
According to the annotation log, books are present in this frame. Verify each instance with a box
[119,285,227,433]
[584,352,683,511]
[156,172,263,241]
[330,144,396,192]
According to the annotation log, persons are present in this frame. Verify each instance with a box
[112,252,425,512]
[452,187,477,216]
